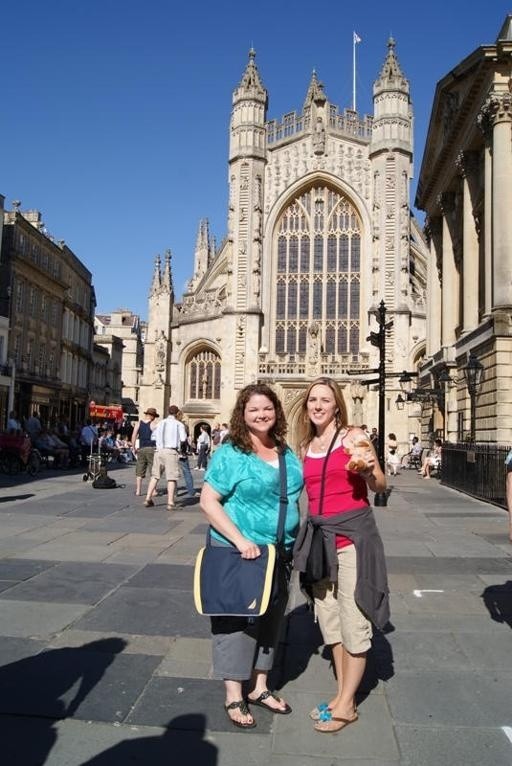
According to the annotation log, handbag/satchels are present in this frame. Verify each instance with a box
[191,544,288,618]
[299,527,328,585]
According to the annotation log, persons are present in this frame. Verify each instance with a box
[283,377,386,732]
[504,448,512,541]
[194,385,303,726]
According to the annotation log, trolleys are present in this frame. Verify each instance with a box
[79,435,110,482]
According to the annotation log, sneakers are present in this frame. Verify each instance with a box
[417,468,425,475]
[194,466,207,471]
[189,491,201,497]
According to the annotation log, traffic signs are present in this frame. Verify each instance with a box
[347,369,380,375]
[366,312,395,348]
[386,372,420,377]
[361,377,382,385]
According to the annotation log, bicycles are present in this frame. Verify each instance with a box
[0,439,42,479]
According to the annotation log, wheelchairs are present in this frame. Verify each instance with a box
[401,451,422,471]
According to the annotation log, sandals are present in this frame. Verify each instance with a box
[167,504,183,510]
[143,499,155,508]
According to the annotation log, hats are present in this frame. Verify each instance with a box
[144,408,159,417]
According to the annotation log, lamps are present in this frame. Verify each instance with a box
[398,371,414,395]
[395,394,406,410]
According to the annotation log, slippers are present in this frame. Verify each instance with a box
[224,702,256,730]
[311,703,357,718]
[315,715,359,735]
[246,690,292,715]
[135,490,164,496]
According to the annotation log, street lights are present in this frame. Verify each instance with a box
[460,350,487,446]
